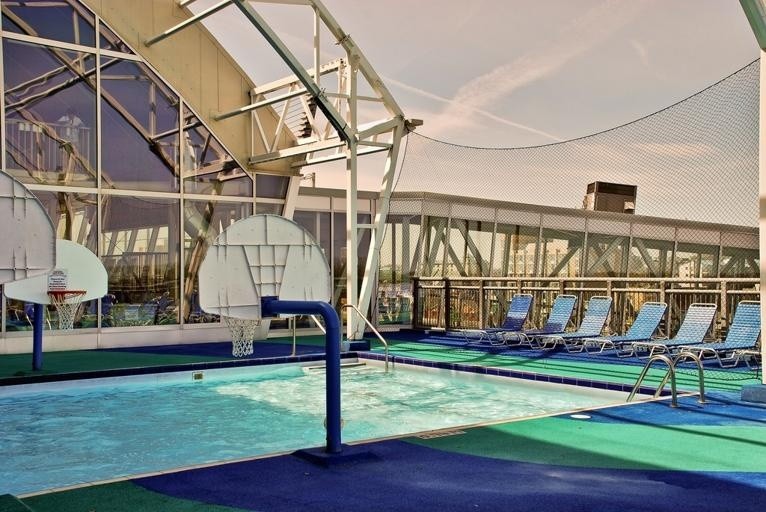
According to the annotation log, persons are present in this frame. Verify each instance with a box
[57,108,85,172]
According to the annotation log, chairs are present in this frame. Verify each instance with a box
[462,293,762,370]
